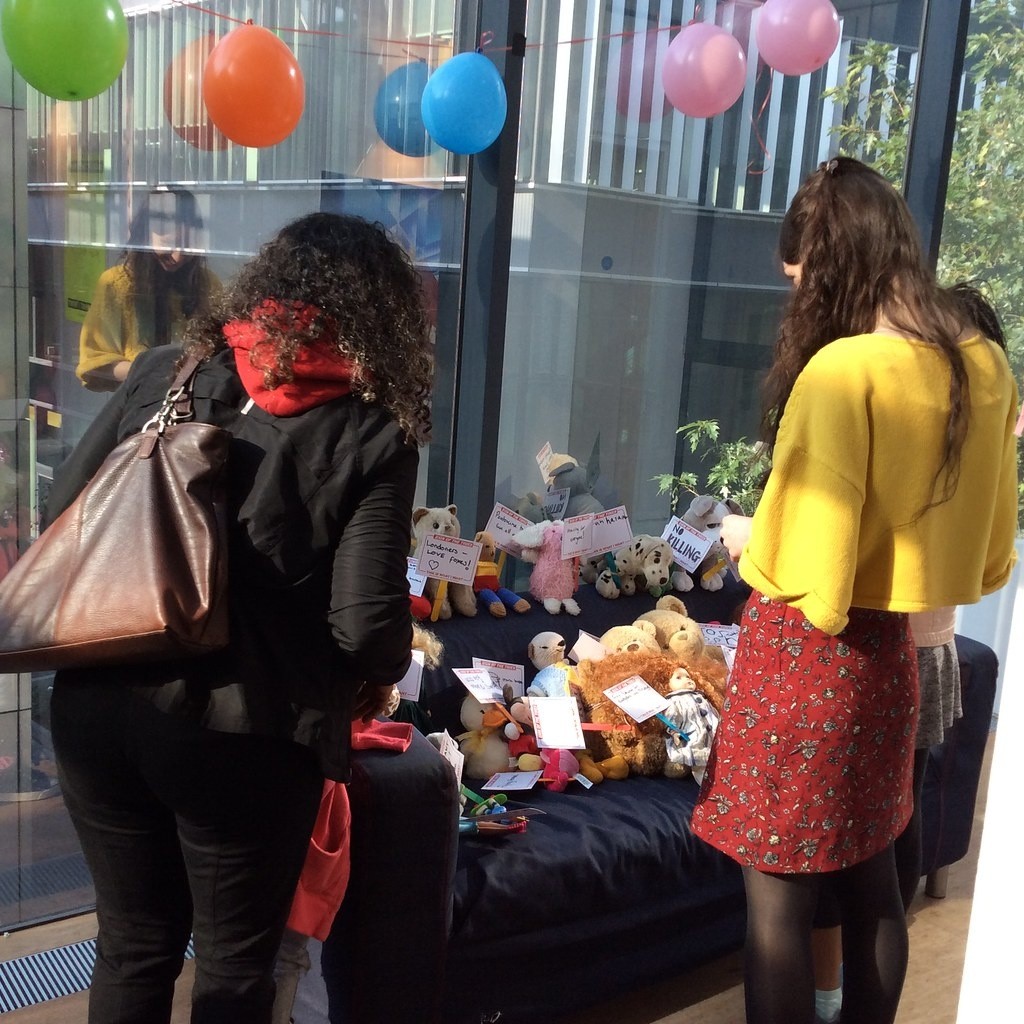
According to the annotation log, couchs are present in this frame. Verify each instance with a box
[282,576,998,1024]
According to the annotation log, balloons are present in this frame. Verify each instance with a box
[662,21,744,118]
[756,0,840,76]
[164,33,236,154]
[0,0,129,101]
[706,0,769,74]
[375,58,443,156]
[204,18,304,147]
[420,45,507,154]
[611,30,675,121]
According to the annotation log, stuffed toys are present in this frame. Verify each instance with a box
[411,453,753,792]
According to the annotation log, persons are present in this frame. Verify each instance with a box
[687,156,1019,1024]
[815,285,1006,1024]
[40,214,432,1024]
[76,183,220,392]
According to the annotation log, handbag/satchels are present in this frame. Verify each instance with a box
[2,347,235,677]
